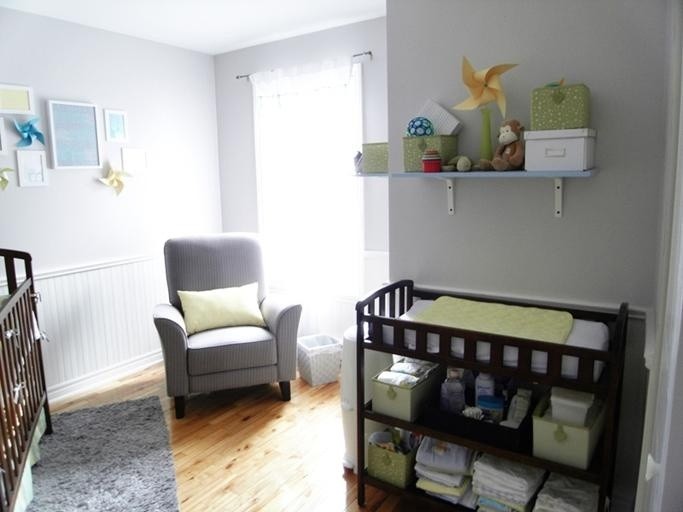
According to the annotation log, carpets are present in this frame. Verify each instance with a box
[17,395,180,512]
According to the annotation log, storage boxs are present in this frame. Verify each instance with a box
[362,142,388,174]
[523,84,598,172]
[403,135,458,172]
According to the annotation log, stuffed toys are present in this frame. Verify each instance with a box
[475,119,525,171]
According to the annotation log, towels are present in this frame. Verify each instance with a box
[376,294,611,384]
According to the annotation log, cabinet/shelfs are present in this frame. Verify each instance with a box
[355,279,630,512]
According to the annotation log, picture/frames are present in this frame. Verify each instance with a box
[0,85,129,188]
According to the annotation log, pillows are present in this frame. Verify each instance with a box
[176,281,268,336]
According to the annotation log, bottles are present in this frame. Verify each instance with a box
[474,372,495,406]
[442,370,465,413]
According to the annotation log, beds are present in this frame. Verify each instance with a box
[0,247,53,512]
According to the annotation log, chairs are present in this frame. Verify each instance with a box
[152,232,302,419]
